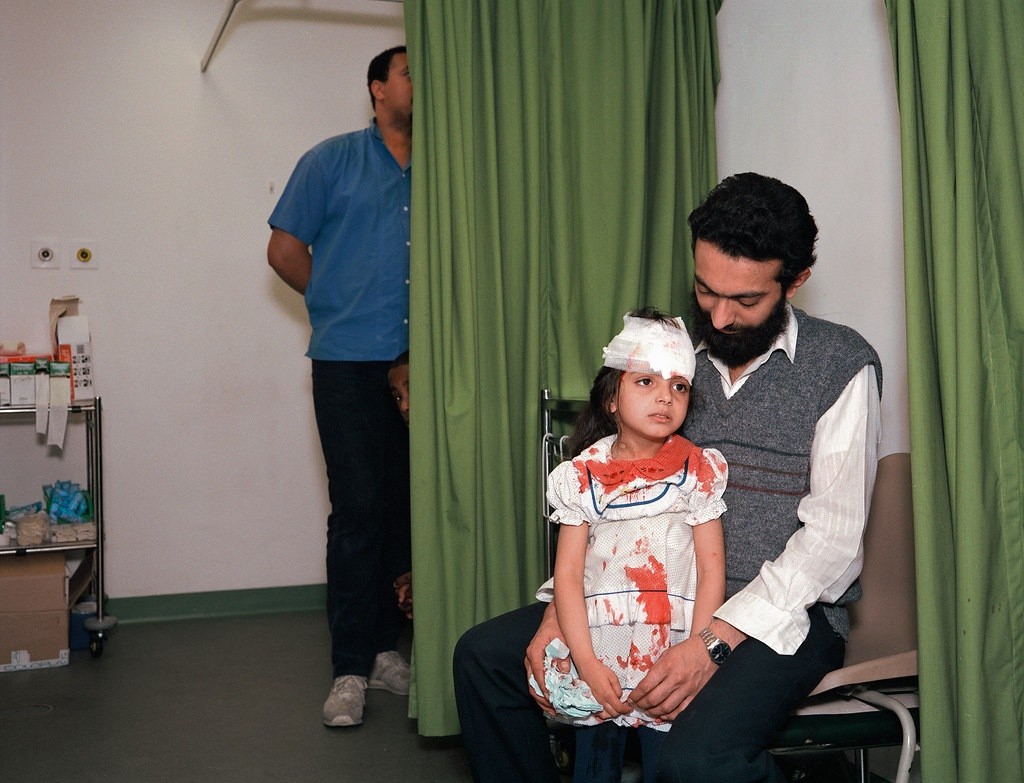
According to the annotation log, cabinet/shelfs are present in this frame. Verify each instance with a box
[0,396,119,658]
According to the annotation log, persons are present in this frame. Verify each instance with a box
[267,45,413,730]
[529,304,730,783]
[451,171,883,782]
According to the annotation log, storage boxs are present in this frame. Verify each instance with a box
[0,296,94,406]
[0,551,97,673]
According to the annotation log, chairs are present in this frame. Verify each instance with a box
[764,454,921,783]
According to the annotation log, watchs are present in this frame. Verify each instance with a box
[698,626,732,666]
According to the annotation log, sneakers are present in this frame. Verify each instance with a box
[367,650,412,695]
[323,674,369,731]
